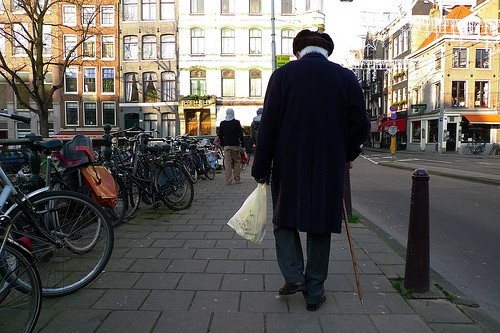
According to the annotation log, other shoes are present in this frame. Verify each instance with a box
[227,179,241,184]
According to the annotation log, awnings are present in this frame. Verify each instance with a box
[462,114,500,124]
[378,118,407,132]
[49,131,104,140]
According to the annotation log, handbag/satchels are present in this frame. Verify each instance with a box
[240,151,249,163]
[227,181,267,246]
[79,165,118,208]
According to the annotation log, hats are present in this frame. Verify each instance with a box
[257,108,264,115]
[226,109,234,117]
[293,29,334,57]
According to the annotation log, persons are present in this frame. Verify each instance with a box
[252,29,370,311]
[219,108,246,184]
[250,108,263,145]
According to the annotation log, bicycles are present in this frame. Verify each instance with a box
[463,139,487,155]
[0,111,225,333]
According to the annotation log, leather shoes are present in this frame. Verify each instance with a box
[279,282,304,295]
[305,294,326,311]
